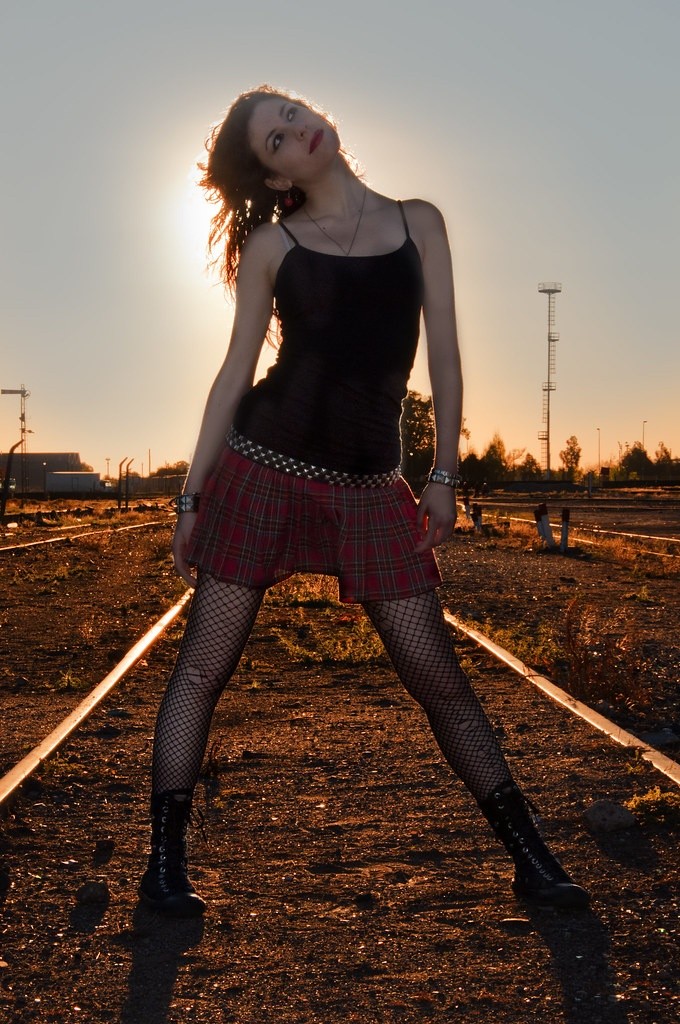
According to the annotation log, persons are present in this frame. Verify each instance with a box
[136,86,594,919]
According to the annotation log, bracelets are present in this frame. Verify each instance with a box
[168,492,203,514]
[423,465,461,490]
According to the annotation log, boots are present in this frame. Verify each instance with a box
[478,790,593,909]
[136,789,207,916]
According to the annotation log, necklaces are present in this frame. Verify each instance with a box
[302,182,367,256]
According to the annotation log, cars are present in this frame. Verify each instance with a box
[0,478,16,499]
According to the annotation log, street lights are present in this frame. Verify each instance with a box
[642,420,649,452]
[41,461,48,495]
[597,428,601,473]
